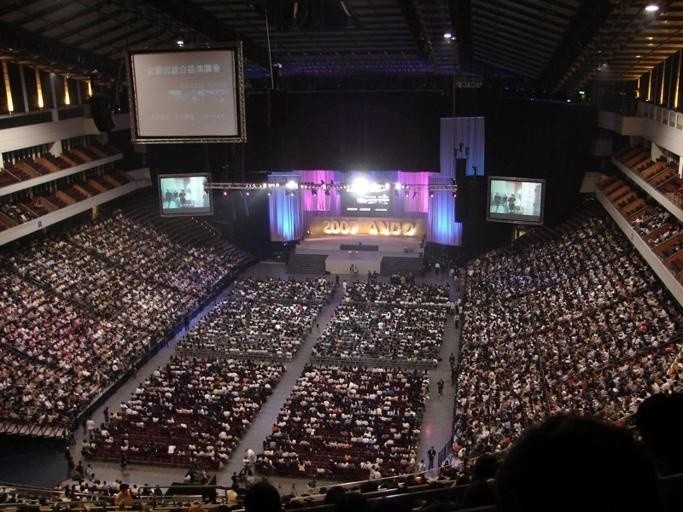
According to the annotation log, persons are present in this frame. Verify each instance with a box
[2,193,683,511]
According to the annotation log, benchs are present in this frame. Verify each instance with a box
[81,413,348,482]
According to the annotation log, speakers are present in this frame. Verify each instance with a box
[88,96,115,133]
